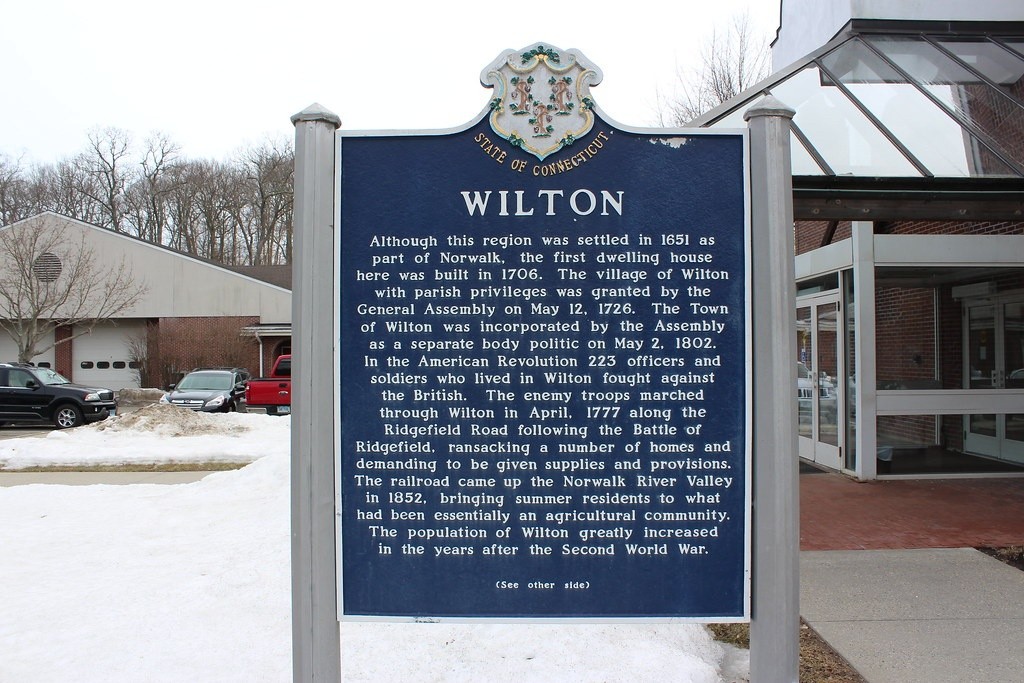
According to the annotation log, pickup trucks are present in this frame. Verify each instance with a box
[244,354,291,415]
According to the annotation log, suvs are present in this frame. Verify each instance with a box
[158,367,253,413]
[797,361,834,422]
[0,363,118,429]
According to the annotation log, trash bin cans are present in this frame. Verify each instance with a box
[876,445,895,474]
